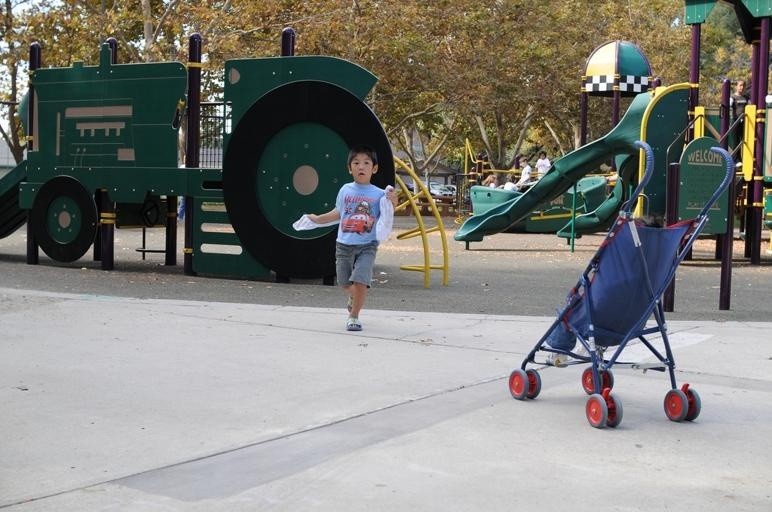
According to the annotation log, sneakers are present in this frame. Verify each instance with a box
[346,295,363,332]
[546,344,593,367]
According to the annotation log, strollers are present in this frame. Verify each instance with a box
[510,141,734,430]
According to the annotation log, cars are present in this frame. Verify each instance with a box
[404,182,457,196]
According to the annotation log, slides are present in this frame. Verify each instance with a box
[0,158,28,239]
[454,90,655,242]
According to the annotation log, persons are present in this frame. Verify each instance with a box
[292,148,400,331]
[503,174,523,192]
[534,151,551,180]
[482,174,498,189]
[515,155,533,185]
[729,81,752,119]
